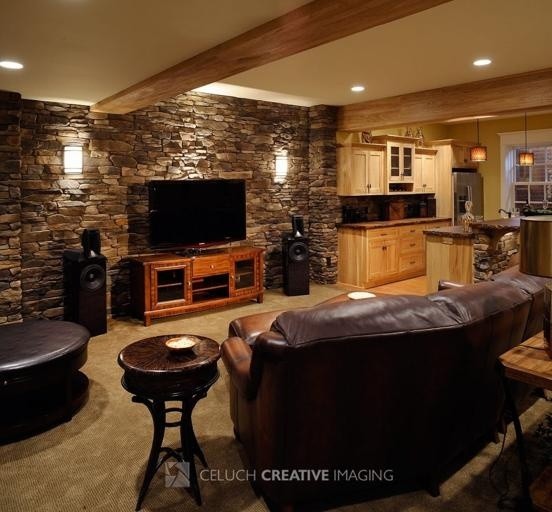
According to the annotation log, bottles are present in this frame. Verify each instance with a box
[406,127,425,148]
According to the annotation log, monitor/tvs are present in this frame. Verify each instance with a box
[151,179,247,248]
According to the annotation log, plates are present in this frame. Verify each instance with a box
[167,336,200,350]
[347,291,376,299]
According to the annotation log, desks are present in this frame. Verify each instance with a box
[117,334,226,512]
[495,327,552,511]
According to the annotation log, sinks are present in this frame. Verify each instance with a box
[496,218,509,221]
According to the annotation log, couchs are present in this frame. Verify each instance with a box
[221,274,550,511]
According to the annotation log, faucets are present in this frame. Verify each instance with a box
[498,209,512,218]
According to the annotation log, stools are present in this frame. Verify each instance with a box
[0,318,91,445]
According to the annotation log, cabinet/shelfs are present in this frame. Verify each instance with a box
[338,225,398,292]
[423,221,450,232]
[124,241,268,328]
[395,224,425,281]
[371,135,417,184]
[404,148,437,195]
[427,140,480,220]
[336,145,385,198]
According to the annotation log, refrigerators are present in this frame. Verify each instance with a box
[452,171,485,226]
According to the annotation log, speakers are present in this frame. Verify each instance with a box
[293,216,305,236]
[281,237,309,295]
[84,229,101,257]
[63,252,107,337]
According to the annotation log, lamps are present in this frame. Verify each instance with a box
[518,112,537,167]
[60,142,84,175]
[273,152,291,181]
[468,116,489,163]
